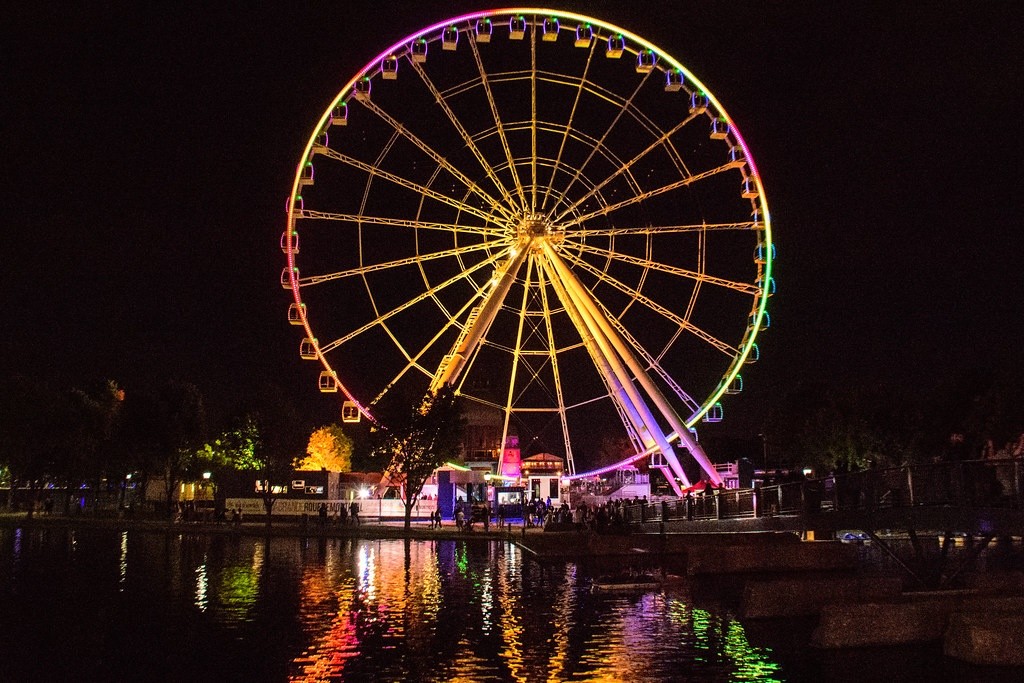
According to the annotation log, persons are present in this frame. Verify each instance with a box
[339,503,348,525]
[435,507,443,528]
[349,502,361,527]
[530,483,728,526]
[428,511,434,529]
[332,510,338,527]
[457,508,464,532]
[319,502,329,524]
[498,504,505,528]
